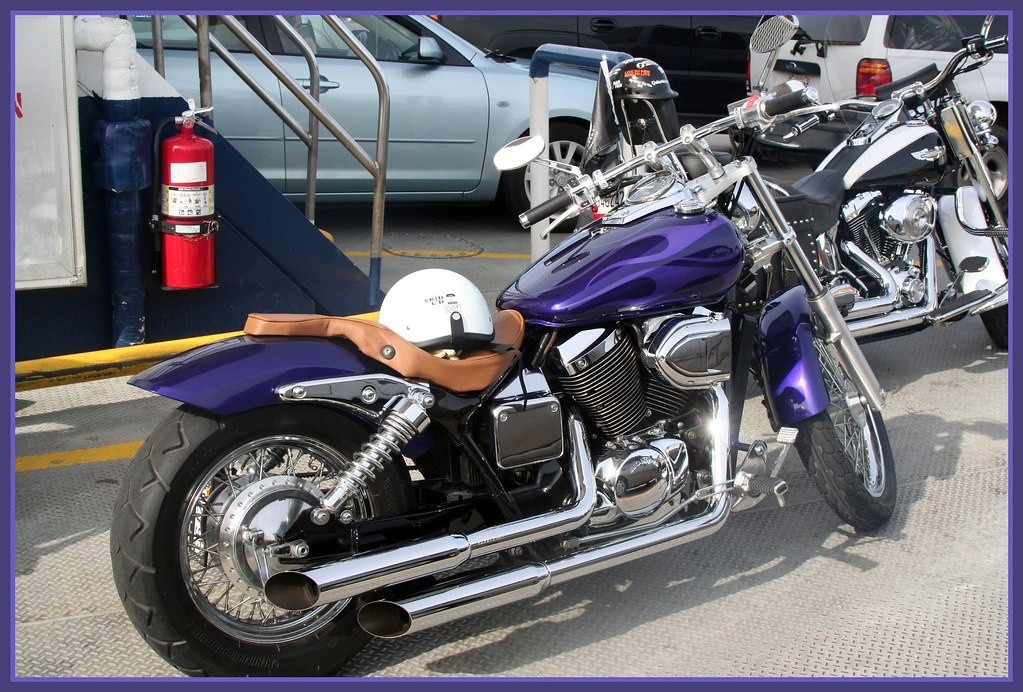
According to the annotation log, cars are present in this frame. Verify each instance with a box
[88,15,632,233]
[744,16,1008,165]
[424,16,869,149]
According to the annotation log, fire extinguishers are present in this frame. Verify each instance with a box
[162,98,215,289]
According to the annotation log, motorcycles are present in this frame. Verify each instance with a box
[106,16,1009,677]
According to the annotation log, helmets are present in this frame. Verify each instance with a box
[379,268,494,349]
[607,57,679,103]
